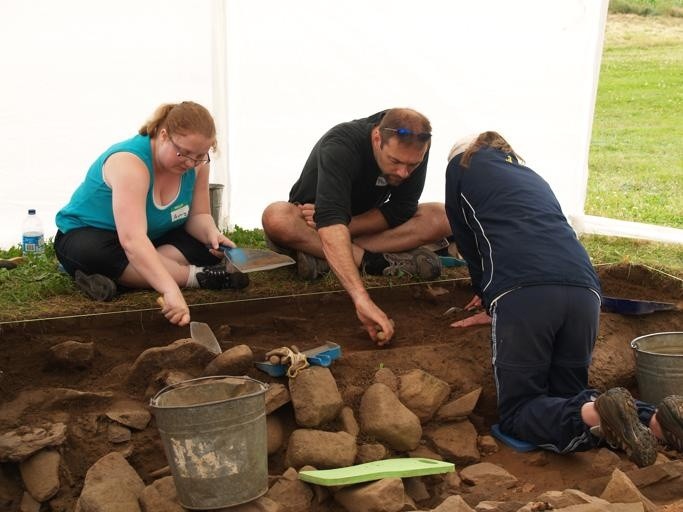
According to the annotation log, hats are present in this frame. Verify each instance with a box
[448,131,481,163]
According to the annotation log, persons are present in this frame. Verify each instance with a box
[53,101,249,326]
[443,130,682,468]
[259,104,456,347]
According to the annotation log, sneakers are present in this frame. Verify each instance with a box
[71,270,117,303]
[195,265,249,292]
[381,247,442,280]
[595,386,658,470]
[654,393,682,452]
[296,252,330,280]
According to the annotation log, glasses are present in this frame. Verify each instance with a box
[166,135,209,168]
[381,125,432,146]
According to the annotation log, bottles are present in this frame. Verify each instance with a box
[19,208,45,257]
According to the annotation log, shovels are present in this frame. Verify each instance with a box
[444,307,464,316]
[375,318,396,340]
[256,341,345,378]
[156,296,222,355]
[221,244,296,276]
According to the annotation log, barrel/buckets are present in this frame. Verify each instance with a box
[630,331,683,406]
[209,184,225,230]
[147,375,269,510]
